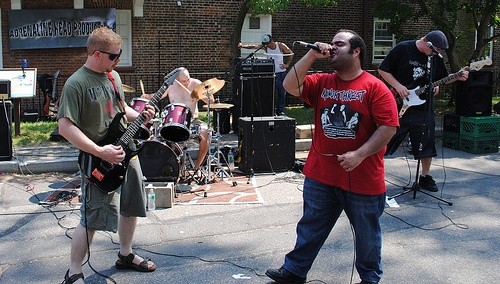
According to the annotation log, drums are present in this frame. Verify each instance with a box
[126,96,155,140]
[137,137,184,185]
[160,103,192,143]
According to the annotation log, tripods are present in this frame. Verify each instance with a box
[388,56,453,207]
[177,89,237,196]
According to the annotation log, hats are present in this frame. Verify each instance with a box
[426,30,449,50]
[262,35,272,45]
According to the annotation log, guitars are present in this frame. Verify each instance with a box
[43,69,62,117]
[389,56,492,118]
[77,68,182,195]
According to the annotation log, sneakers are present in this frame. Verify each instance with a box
[265,266,305,284]
[419,175,438,192]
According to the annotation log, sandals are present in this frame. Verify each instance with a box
[62,269,85,284]
[116,251,156,272]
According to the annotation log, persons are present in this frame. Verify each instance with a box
[238,35,294,116]
[377,30,469,192]
[57,26,155,284]
[265,28,399,284]
[141,67,214,181]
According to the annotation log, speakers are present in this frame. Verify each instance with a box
[0,101,12,160]
[456,69,492,117]
[233,75,274,131]
[238,115,296,172]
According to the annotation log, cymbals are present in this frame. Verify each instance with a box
[203,103,235,110]
[191,77,225,100]
[122,83,135,92]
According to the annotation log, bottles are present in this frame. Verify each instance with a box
[148,189,156,211]
[228,150,234,171]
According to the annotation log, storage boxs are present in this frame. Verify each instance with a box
[144,181,174,208]
[296,125,315,138]
[443,112,500,154]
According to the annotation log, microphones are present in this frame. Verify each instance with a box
[300,41,333,55]
[426,42,443,59]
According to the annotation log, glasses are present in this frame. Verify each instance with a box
[92,49,122,61]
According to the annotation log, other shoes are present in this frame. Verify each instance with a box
[279,112,285,115]
